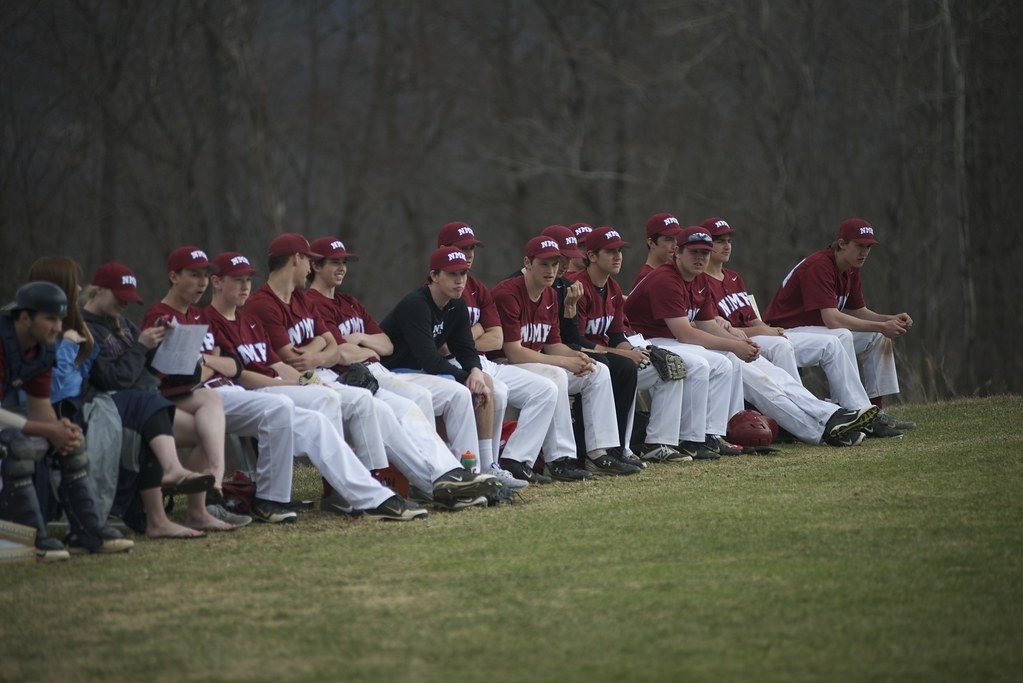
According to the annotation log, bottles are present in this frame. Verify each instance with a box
[461,450,477,474]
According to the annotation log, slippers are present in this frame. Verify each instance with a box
[162,472,215,493]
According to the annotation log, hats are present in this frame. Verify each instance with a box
[646,213,684,237]
[700,218,735,236]
[540,224,587,259]
[585,227,631,251]
[309,237,359,262]
[526,236,563,260]
[839,219,880,246]
[92,262,143,304]
[168,246,220,273]
[677,225,717,253]
[437,222,484,250]
[211,252,255,276]
[567,223,594,244]
[267,232,325,258]
[430,245,470,272]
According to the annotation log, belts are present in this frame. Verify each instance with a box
[208,377,231,388]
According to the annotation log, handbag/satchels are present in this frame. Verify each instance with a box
[336,362,380,396]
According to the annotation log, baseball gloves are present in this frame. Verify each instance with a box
[336,362,380,396]
[299,368,323,386]
[646,344,687,381]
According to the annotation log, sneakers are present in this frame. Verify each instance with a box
[65,537,132,554]
[205,430,742,534]
[36,548,69,562]
[823,405,916,446]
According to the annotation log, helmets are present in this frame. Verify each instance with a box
[0,282,67,313]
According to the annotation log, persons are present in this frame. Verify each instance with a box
[624,226,761,460]
[0,282,136,560]
[380,247,529,489]
[244,233,503,508]
[78,263,236,529]
[507,225,644,469]
[490,236,641,482]
[700,217,903,439]
[26,255,216,538]
[764,218,917,430]
[565,223,693,468]
[140,247,429,525]
[424,222,558,484]
[632,212,880,446]
[304,237,481,475]
